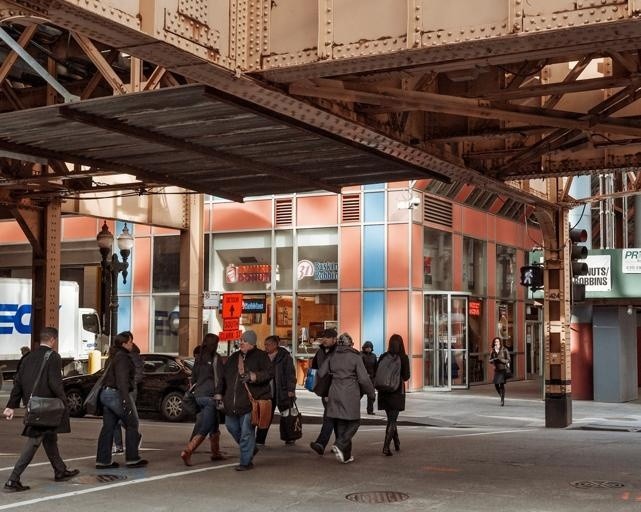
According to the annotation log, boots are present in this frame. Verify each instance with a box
[180,434,205,466]
[500,395,505,406]
[209,431,230,461]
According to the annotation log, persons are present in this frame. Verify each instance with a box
[354,342,377,415]
[488,337,511,406]
[180,332,297,471]
[2,327,79,491]
[316,333,368,466]
[16,347,32,408]
[111,332,143,454]
[310,329,342,456]
[375,334,411,456]
[95,331,149,469]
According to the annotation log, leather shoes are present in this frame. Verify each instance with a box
[54,468,80,481]
[3,480,29,493]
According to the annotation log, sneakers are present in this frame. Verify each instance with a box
[310,441,324,455]
[383,448,394,457]
[95,459,120,470]
[394,444,400,451]
[331,444,354,464]
[111,447,124,455]
[234,461,255,471]
[284,440,296,446]
[126,460,148,470]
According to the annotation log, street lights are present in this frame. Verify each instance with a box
[94,220,137,363]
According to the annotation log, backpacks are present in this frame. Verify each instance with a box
[374,352,404,391]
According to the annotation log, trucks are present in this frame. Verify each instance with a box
[1,273,104,393]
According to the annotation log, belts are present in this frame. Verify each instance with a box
[102,385,121,394]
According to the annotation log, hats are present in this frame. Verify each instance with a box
[321,328,337,338]
[241,329,257,346]
[335,333,354,346]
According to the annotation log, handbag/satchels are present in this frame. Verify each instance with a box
[279,412,300,440]
[82,384,106,416]
[182,390,202,417]
[315,372,333,396]
[305,367,319,392]
[504,364,513,379]
[214,387,226,414]
[250,399,272,429]
[23,395,65,429]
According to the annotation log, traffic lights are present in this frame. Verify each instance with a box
[518,262,546,287]
[568,221,590,276]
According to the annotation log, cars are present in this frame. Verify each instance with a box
[61,351,226,428]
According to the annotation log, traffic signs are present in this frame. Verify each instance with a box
[219,329,243,341]
[222,292,242,319]
[223,317,240,331]
